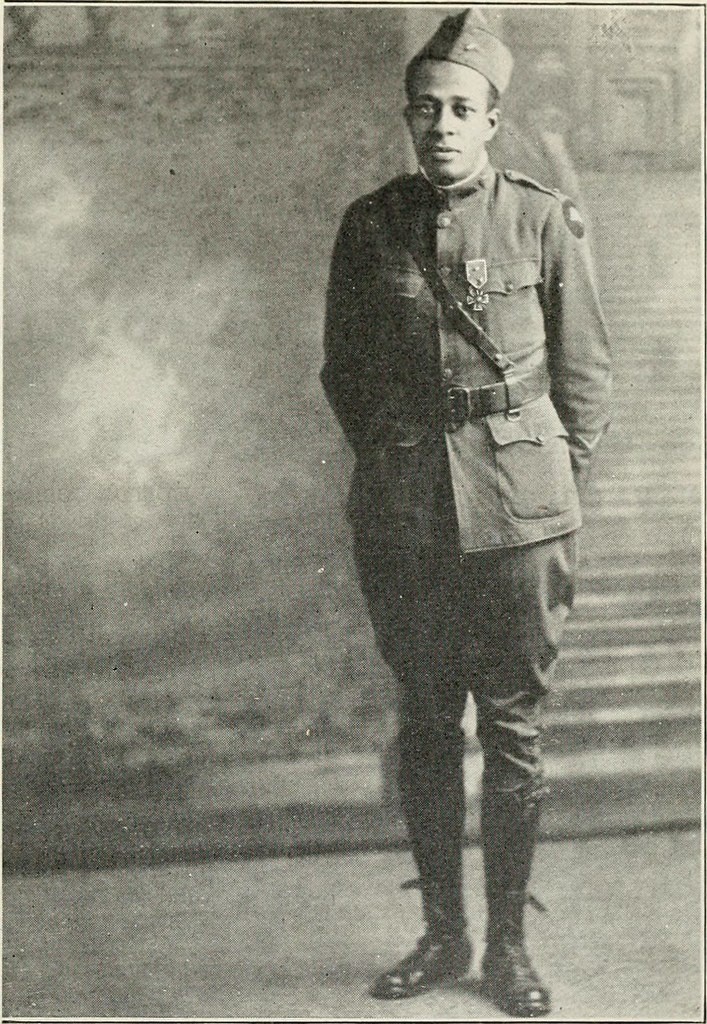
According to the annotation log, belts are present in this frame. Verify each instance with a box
[372,361,548,416]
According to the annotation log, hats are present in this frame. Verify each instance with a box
[413,7,514,96]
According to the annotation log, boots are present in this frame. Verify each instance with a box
[483,824,549,1015]
[369,824,471,998]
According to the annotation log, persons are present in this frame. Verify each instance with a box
[318,33,613,1017]
